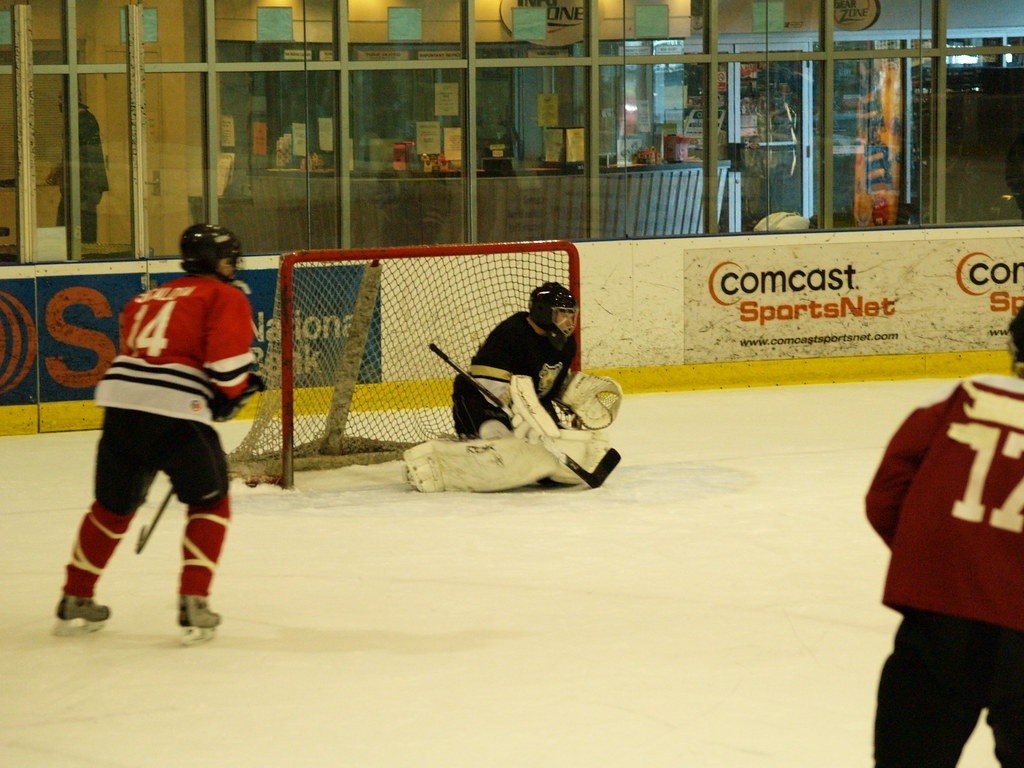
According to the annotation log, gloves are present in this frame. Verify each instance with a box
[207,372,267,421]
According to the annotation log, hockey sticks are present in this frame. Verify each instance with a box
[134,488,174,557]
[429,343,623,490]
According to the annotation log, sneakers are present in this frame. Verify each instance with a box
[179,594,220,645]
[53,591,110,638]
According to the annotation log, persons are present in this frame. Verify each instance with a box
[47,89,110,242]
[452,284,576,484]
[50,224,255,646]
[865,306,1024,768]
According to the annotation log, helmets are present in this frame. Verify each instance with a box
[180,223,241,273]
[528,281,577,329]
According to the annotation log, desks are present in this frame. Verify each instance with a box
[0,243,154,263]
